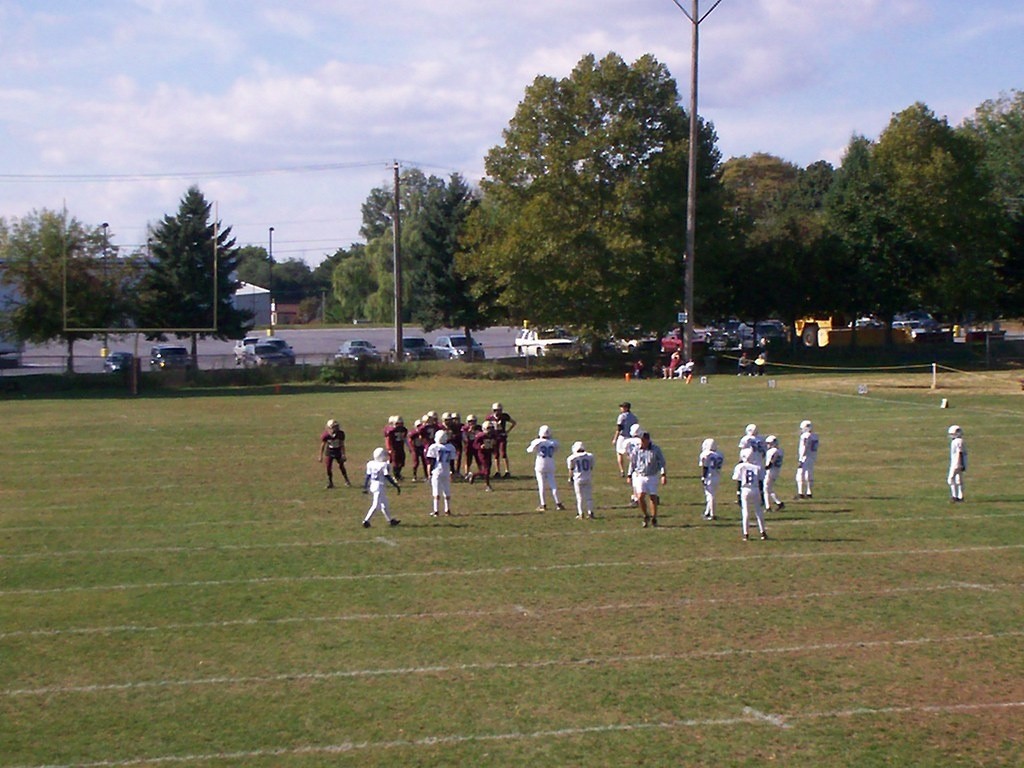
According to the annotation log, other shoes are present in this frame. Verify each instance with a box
[323,470,813,541]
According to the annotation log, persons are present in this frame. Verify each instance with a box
[795,419,819,498]
[699,438,724,521]
[612,402,667,527]
[473,421,499,492]
[947,424,967,502]
[566,441,595,520]
[732,424,784,542]
[363,447,401,528]
[634,351,694,380]
[737,337,767,377]
[383,402,517,484]
[526,425,567,511]
[426,430,455,517]
[318,419,352,489]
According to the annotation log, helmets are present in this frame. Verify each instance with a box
[415,419,421,427]
[800,420,812,432]
[746,423,757,435]
[393,415,403,424]
[572,441,584,451]
[740,448,754,462]
[766,435,779,446]
[442,412,451,419]
[374,448,385,461]
[539,425,551,438]
[467,414,477,421]
[492,402,501,410]
[428,410,438,418]
[949,425,960,434]
[434,430,447,443]
[482,421,493,432]
[738,435,755,448]
[630,424,641,437]
[451,413,460,419]
[389,416,393,423]
[702,438,716,450]
[423,415,430,421]
[327,419,339,428]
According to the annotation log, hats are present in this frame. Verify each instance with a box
[619,402,630,408]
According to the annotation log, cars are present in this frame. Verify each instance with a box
[513,328,574,359]
[793,307,948,348]
[611,316,787,355]
[387,335,438,363]
[233,336,296,369]
[332,338,382,365]
[102,351,133,375]
[149,344,192,369]
[432,334,486,362]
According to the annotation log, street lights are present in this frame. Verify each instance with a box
[101,222,109,348]
[268,227,275,336]
